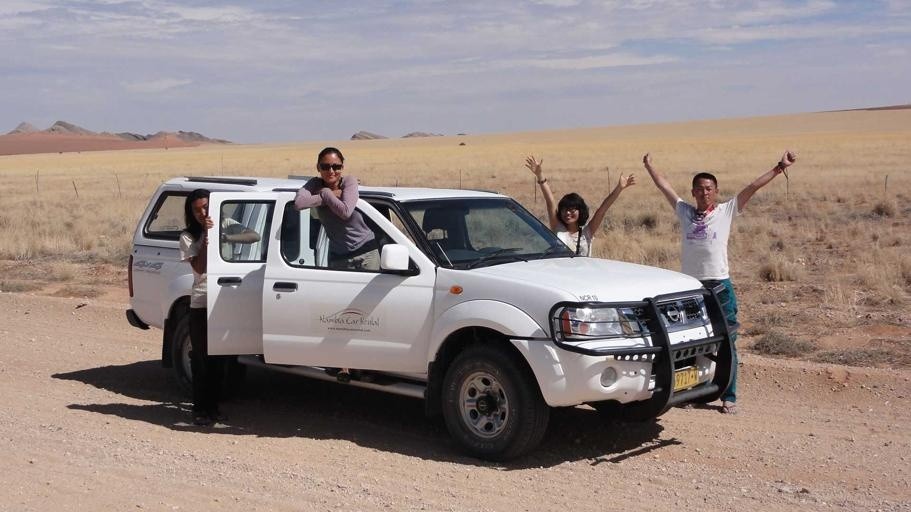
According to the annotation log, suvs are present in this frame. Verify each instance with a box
[127,176,736,461]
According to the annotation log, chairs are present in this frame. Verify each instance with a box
[423,207,466,254]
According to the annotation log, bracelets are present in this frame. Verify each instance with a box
[778,162,788,176]
[538,178,546,185]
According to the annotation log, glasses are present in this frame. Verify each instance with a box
[561,207,578,214]
[320,162,343,171]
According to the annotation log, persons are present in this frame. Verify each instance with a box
[526,153,636,259]
[179,188,262,425]
[643,146,798,416]
[292,146,382,382]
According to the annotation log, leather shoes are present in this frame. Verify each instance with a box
[337,371,351,383]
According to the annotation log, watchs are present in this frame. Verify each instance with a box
[221,232,227,242]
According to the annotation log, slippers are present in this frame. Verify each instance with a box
[722,404,737,414]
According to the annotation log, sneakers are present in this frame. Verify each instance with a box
[191,405,229,426]
